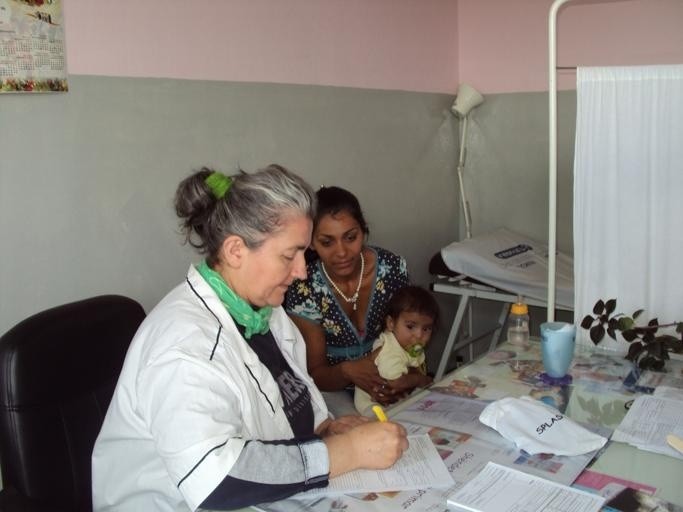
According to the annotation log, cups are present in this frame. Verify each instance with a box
[540,321,577,381]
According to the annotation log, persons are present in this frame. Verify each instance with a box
[352,285,440,417]
[88,162,410,512]
[280,185,409,421]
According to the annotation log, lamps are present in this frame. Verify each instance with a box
[451,82,485,367]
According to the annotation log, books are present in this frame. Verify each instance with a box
[445,459,608,511]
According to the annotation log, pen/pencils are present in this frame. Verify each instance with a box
[372,405,387,423]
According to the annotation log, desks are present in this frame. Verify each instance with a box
[195,335,683,512]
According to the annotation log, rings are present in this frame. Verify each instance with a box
[382,385,385,389]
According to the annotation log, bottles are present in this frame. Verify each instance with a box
[506,293,532,348]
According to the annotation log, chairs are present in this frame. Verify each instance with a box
[0,294,147,512]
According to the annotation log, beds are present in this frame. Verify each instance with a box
[429,225,574,383]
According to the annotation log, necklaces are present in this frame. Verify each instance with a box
[321,252,366,311]
